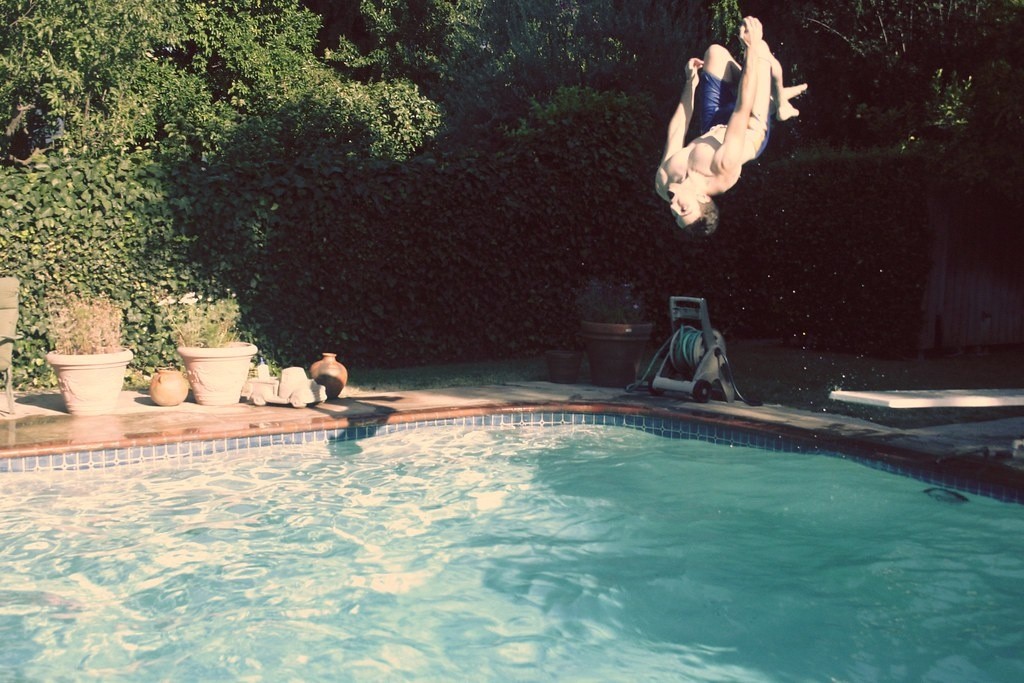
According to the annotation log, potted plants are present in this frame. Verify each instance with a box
[580,300,651,390]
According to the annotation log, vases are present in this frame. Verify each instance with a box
[176,341,258,405]
[45,351,132,414]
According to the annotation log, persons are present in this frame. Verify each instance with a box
[655,15,807,239]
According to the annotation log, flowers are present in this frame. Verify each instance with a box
[156,287,244,348]
[49,289,130,353]
